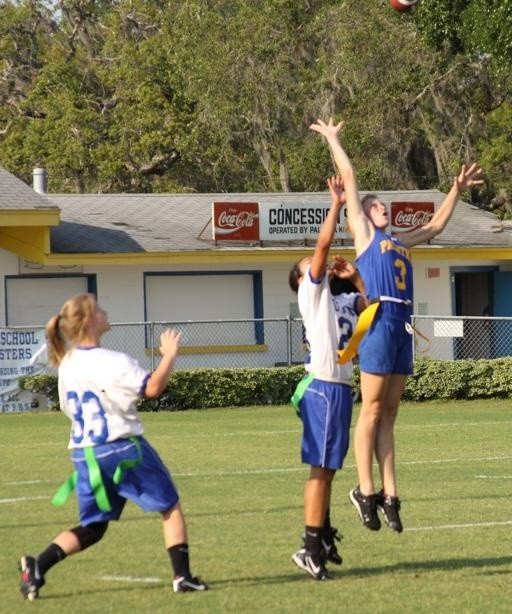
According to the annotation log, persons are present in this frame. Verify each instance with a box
[16,291,211,602]
[288,174,368,584]
[309,117,485,532]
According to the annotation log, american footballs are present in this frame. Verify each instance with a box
[391,0,418,11]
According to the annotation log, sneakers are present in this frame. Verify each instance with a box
[172,576,208,593]
[302,526,341,565]
[349,486,382,529]
[291,549,332,580]
[18,555,45,599]
[378,488,403,533]
